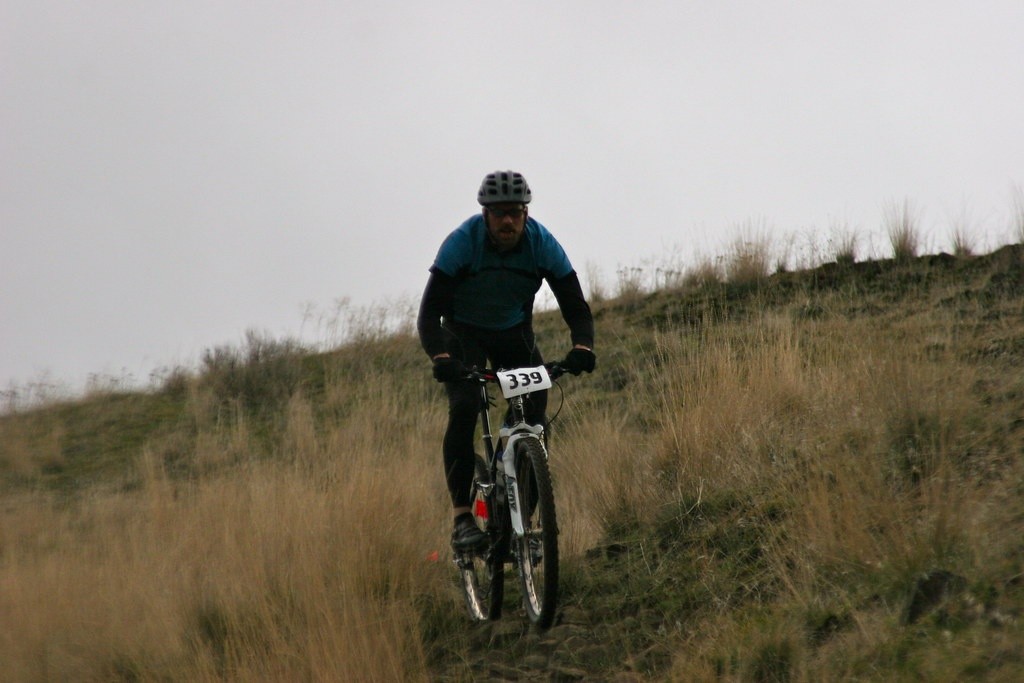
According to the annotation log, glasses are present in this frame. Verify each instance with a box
[486,207,524,218]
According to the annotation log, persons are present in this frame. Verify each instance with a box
[417,171,597,567]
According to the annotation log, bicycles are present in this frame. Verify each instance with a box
[450,356,602,632]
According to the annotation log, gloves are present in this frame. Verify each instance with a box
[432,357,465,382]
[565,348,595,376]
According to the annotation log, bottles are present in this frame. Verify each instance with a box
[496,451,505,503]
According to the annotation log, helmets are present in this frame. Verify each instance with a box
[477,170,531,205]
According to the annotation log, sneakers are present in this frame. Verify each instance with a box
[508,522,542,564]
[450,520,489,553]
